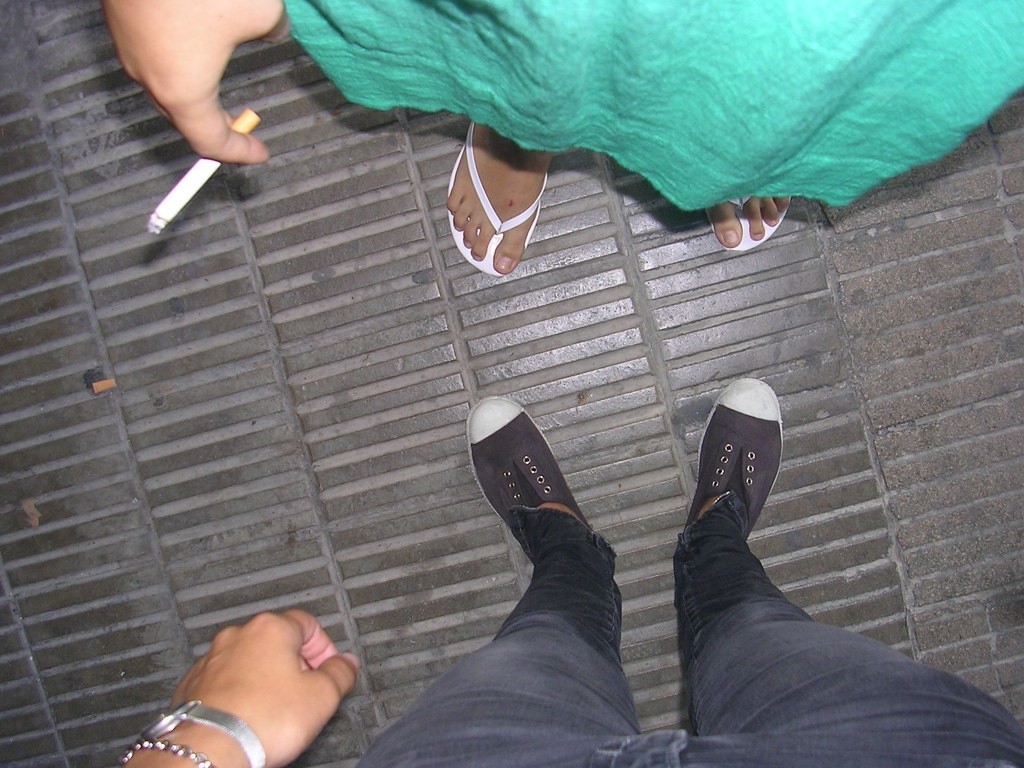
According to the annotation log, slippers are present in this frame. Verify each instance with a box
[706,193,791,251]
[445,120,547,279]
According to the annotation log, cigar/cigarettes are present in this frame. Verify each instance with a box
[147,108,262,235]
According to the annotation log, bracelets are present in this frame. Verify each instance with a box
[117,738,212,768]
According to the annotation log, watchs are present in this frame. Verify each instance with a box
[134,698,267,768]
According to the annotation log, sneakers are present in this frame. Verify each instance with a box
[683,378,783,542]
[466,397,594,568]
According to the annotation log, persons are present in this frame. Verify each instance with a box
[98,0,1024,280]
[114,378,1024,768]
